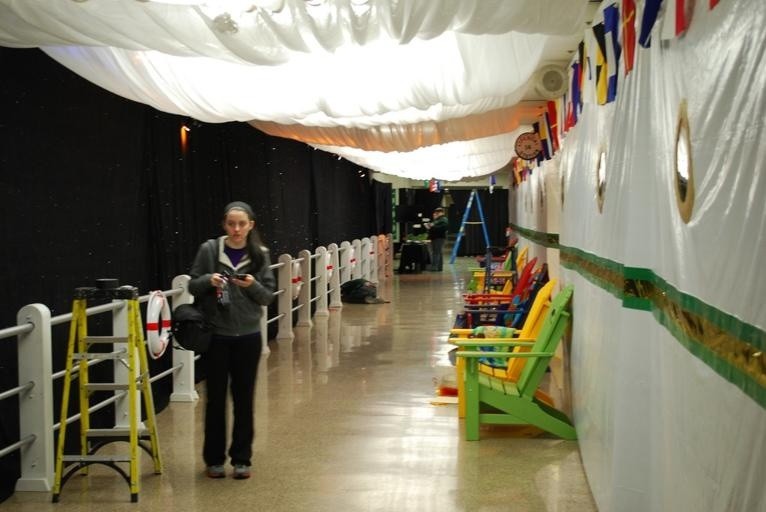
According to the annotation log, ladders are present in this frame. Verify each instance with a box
[52,285,163,502]
[449,187,491,263]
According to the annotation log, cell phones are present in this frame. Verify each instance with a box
[236,273,247,280]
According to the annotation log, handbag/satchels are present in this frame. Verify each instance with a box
[171,304,205,350]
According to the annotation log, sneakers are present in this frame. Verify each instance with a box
[233,464,250,478]
[206,465,225,477]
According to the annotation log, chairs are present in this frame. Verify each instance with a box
[451,237,576,442]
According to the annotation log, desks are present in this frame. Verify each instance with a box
[400,242,431,273]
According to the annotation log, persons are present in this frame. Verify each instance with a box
[188,201,276,479]
[426,208,448,271]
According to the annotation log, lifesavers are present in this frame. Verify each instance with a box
[370,243,374,261]
[350,249,355,272]
[326,253,333,284]
[146,291,171,358]
[292,263,302,299]
